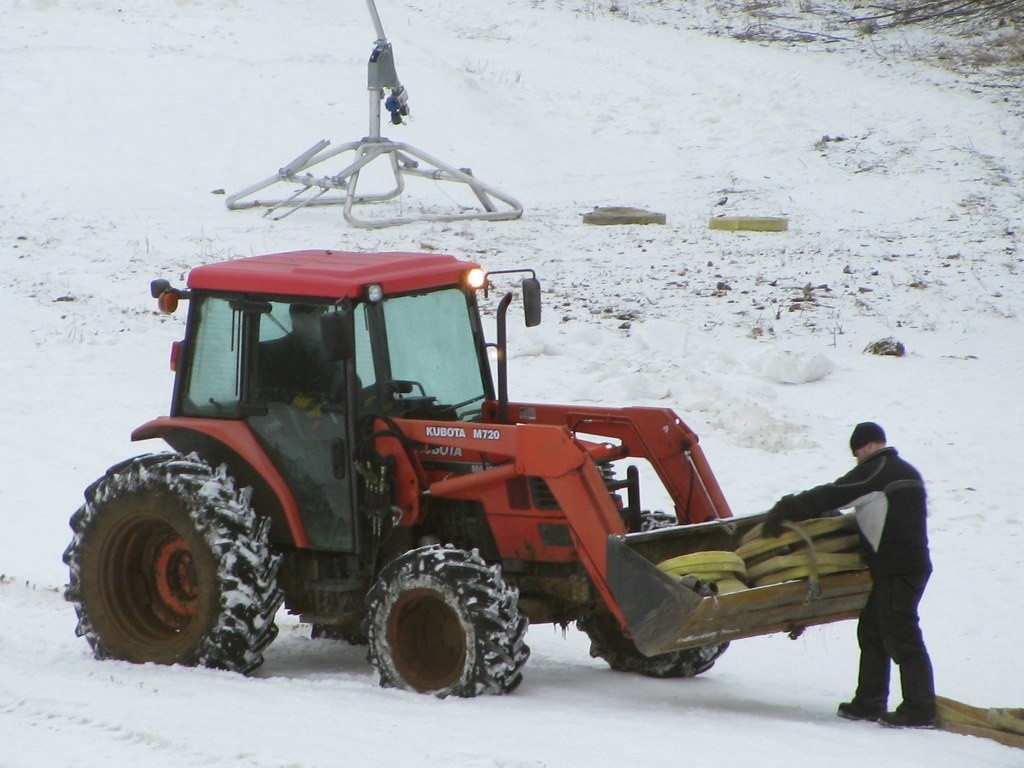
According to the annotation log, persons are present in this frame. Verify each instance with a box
[760,422,936,729]
[256,305,362,414]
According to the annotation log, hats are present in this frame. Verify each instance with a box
[850,422,886,451]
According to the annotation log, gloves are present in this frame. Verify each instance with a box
[761,501,787,539]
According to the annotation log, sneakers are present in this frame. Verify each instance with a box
[877,711,933,729]
[837,703,887,719]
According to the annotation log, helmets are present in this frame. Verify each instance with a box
[289,303,329,318]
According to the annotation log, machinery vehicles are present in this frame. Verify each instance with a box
[57,245,875,705]
